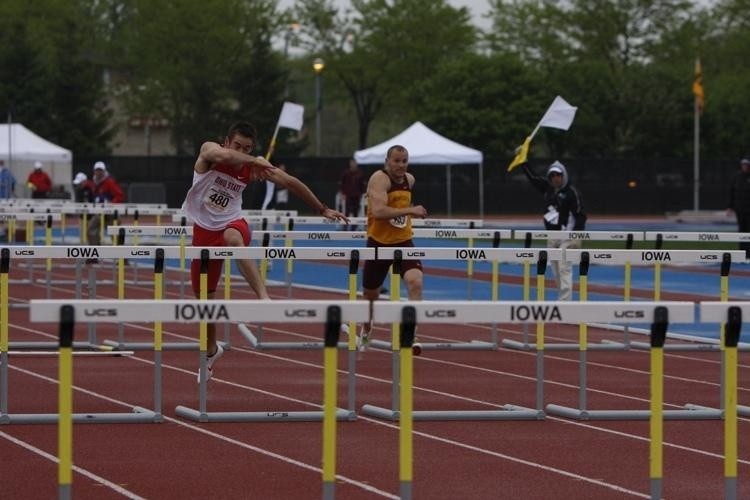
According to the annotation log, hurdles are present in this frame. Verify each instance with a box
[360,245,564,422]
[0,243,184,426]
[29,296,372,498]
[234,230,368,350]
[174,246,377,422]
[545,247,748,422]
[372,298,696,498]
[1,197,196,308]
[368,229,513,354]
[502,231,645,351]
[215,209,370,296]
[410,218,485,276]
[700,301,750,499]
[101,225,231,351]
[631,230,749,353]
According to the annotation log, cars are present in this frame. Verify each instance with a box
[439,171,538,185]
[626,169,694,193]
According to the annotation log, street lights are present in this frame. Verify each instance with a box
[313,57,325,157]
[283,21,302,97]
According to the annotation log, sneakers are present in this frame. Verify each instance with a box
[411,337,421,356]
[359,326,373,352]
[198,344,224,384]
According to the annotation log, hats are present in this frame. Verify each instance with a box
[547,166,562,176]
[94,161,106,171]
[73,172,87,185]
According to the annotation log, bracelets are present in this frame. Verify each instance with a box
[319,203,328,215]
[249,155,256,166]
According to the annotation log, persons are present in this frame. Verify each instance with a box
[72,172,91,195]
[357,144,429,354]
[27,159,54,199]
[87,161,127,265]
[725,158,749,262]
[515,147,589,310]
[181,122,351,384]
[0,156,16,199]
[340,159,366,232]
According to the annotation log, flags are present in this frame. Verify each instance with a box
[539,94,577,132]
[278,98,304,133]
[690,60,705,112]
